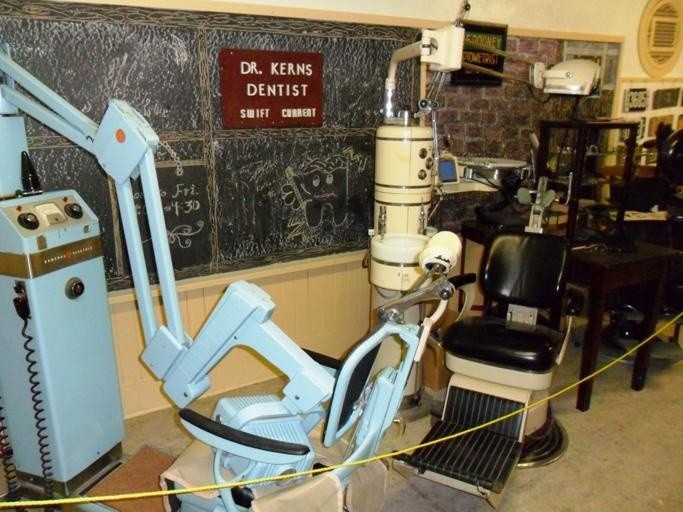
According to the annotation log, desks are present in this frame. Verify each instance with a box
[533,116,642,246]
[565,236,682,412]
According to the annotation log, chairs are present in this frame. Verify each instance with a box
[389,228,581,509]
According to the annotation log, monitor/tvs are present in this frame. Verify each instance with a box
[427,26,466,73]
[438,156,460,185]
[451,19,508,87]
[543,58,602,95]
